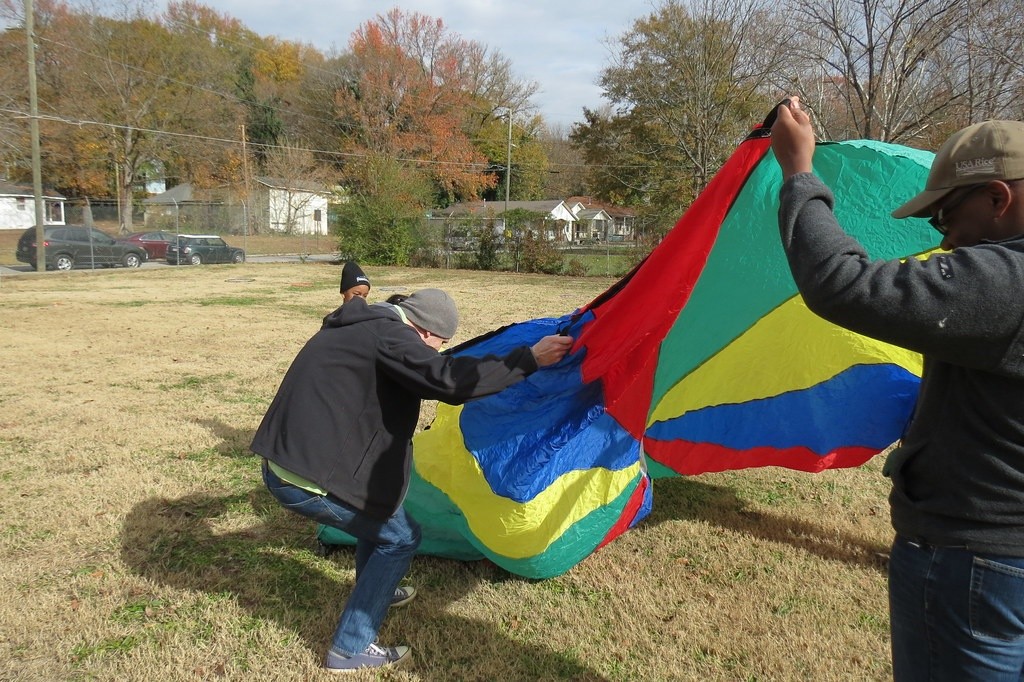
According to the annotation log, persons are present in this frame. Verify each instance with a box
[768,94,1024,682]
[338,260,372,304]
[383,293,439,434]
[248,287,576,672]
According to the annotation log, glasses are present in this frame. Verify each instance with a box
[928,183,1010,236]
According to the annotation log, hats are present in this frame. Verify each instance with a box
[399,288,458,339]
[891,118,1024,218]
[340,262,370,294]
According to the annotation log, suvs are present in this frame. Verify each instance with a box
[16,224,149,271]
[166,233,247,266]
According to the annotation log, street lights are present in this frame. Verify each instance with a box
[490,110,513,224]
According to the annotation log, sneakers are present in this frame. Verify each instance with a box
[325,635,412,674]
[391,586,417,607]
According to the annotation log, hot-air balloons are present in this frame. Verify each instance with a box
[310,103,958,584]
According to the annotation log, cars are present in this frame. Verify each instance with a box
[114,231,175,262]
